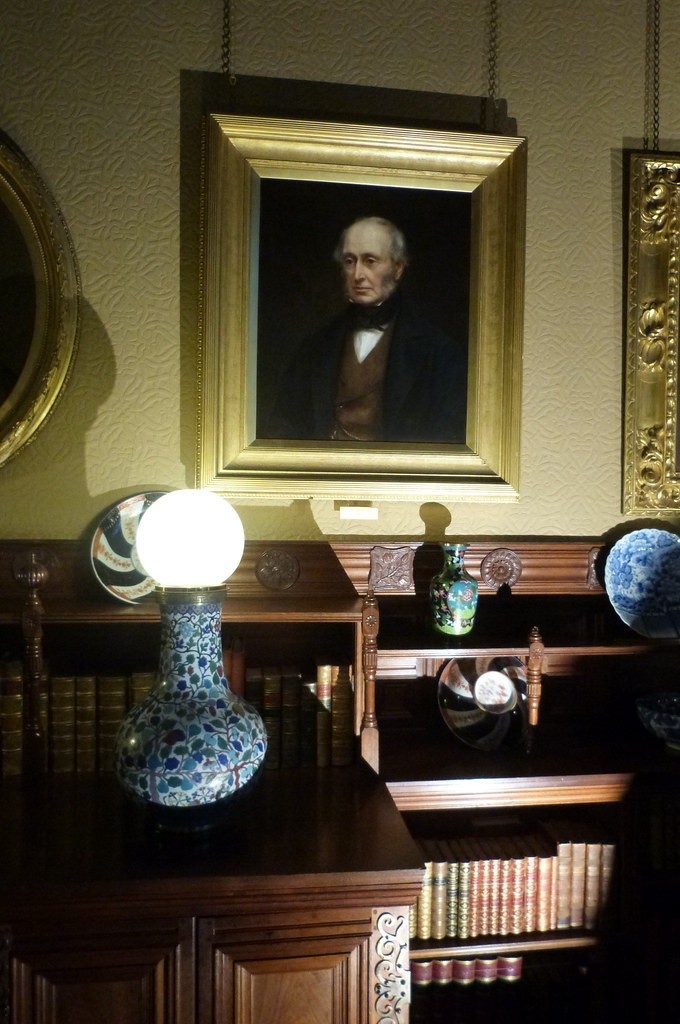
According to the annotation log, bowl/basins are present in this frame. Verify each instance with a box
[635,692,680,750]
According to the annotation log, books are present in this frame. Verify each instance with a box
[409,815,618,942]
[408,954,523,1023]
[0,635,366,780]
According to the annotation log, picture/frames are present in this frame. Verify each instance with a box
[1,128,84,470]
[621,152,680,520]
[196,109,530,501]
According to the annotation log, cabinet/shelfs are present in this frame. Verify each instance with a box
[0,540,680,1024]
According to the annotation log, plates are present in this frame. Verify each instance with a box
[89,491,171,605]
[604,528,680,639]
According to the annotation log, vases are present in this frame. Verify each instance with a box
[113,585,270,838]
[431,543,479,637]
